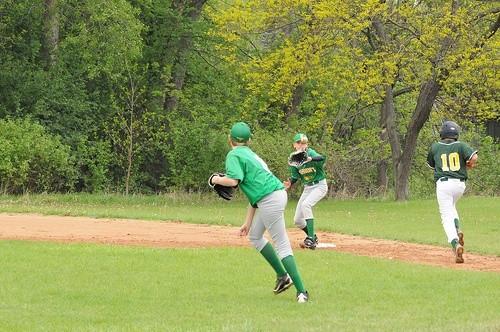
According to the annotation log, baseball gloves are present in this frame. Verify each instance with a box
[287,151,309,167]
[208,172,239,200]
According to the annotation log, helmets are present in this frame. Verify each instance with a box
[439,121,460,141]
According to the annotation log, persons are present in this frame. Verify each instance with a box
[426,121,478,263]
[208,121,309,303]
[283,134,328,250]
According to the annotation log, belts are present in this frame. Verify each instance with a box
[440,178,464,182]
[307,180,319,186]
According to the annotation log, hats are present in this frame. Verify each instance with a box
[293,134,308,143]
[230,122,250,143]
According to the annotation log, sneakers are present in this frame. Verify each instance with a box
[457,229,464,245]
[454,244,464,263]
[304,237,313,248]
[312,234,319,249]
[296,291,309,303]
[273,273,293,294]
